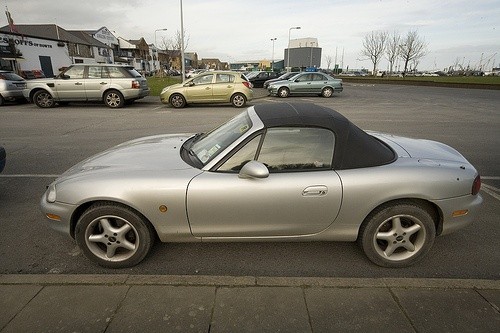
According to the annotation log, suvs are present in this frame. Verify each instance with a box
[25,61,150,108]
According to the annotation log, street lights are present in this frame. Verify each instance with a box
[288,27,302,70]
[270,38,277,71]
[310,41,315,69]
[154,27,167,74]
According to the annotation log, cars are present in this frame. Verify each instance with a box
[263,72,301,90]
[337,67,500,78]
[164,67,285,88]
[160,71,255,108]
[269,71,343,100]
[0,69,26,106]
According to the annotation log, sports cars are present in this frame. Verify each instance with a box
[39,102,483,268]
